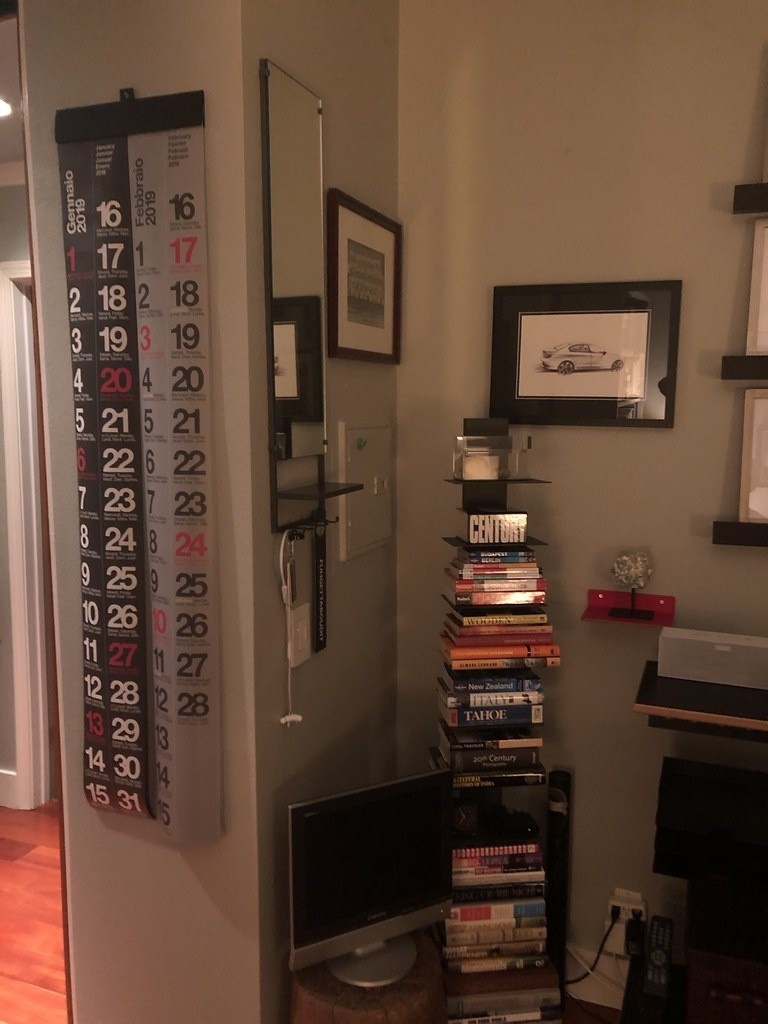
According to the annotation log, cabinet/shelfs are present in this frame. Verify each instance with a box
[428,419,563,1024]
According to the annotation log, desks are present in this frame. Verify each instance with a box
[633,660,768,744]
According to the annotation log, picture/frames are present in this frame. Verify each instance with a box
[745,218,768,356]
[327,187,403,365]
[272,296,324,422]
[489,279,683,429]
[739,388,768,523]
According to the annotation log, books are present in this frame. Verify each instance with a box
[426,548,565,774]
[432,838,563,1023]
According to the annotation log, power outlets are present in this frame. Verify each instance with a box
[607,895,647,922]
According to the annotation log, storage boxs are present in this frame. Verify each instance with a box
[657,627,768,690]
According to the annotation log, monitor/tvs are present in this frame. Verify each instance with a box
[286,767,451,989]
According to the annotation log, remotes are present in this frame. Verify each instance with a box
[642,915,674,998]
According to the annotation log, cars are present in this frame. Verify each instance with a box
[538,342,626,375]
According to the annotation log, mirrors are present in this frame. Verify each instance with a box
[261,57,327,463]
